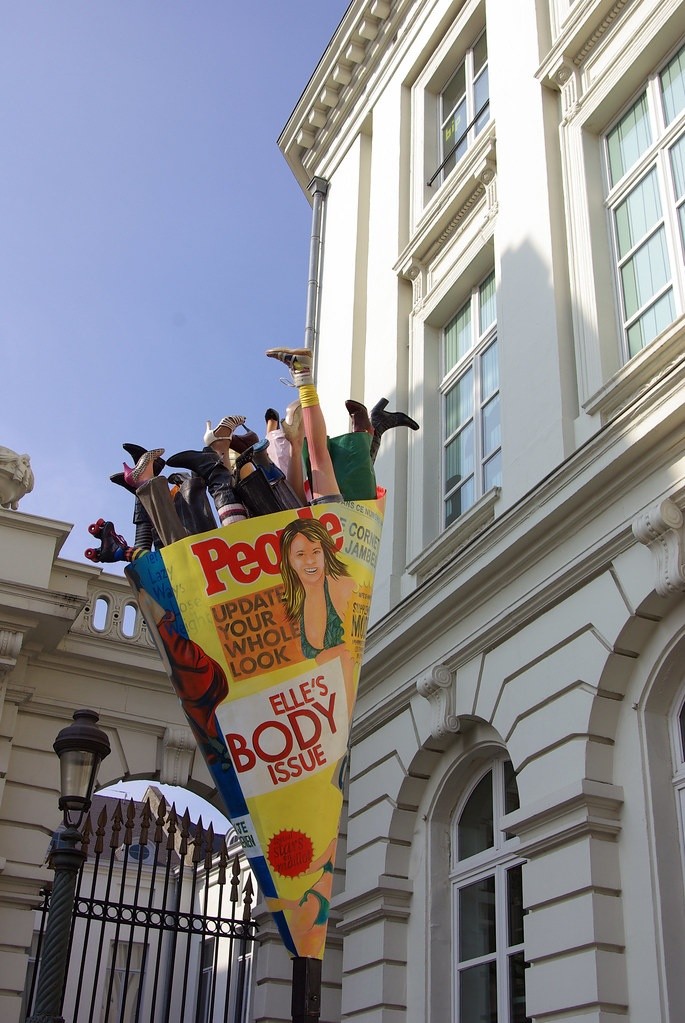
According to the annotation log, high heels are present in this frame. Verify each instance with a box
[123,448,165,488]
[229,424,259,454]
[204,415,247,446]
[168,471,200,485]
[232,446,253,486]
[281,398,303,441]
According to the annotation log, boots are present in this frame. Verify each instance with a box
[252,439,285,487]
[369,398,419,439]
[166,446,243,511]
[345,400,374,437]
[110,443,166,524]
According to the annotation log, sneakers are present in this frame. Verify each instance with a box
[266,347,313,387]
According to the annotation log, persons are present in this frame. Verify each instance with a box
[99,346,419,563]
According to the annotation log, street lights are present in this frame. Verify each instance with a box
[23,707,112,1023]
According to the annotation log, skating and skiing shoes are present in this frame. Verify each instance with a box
[84,518,127,563]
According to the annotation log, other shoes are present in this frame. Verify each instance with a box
[265,408,280,430]
